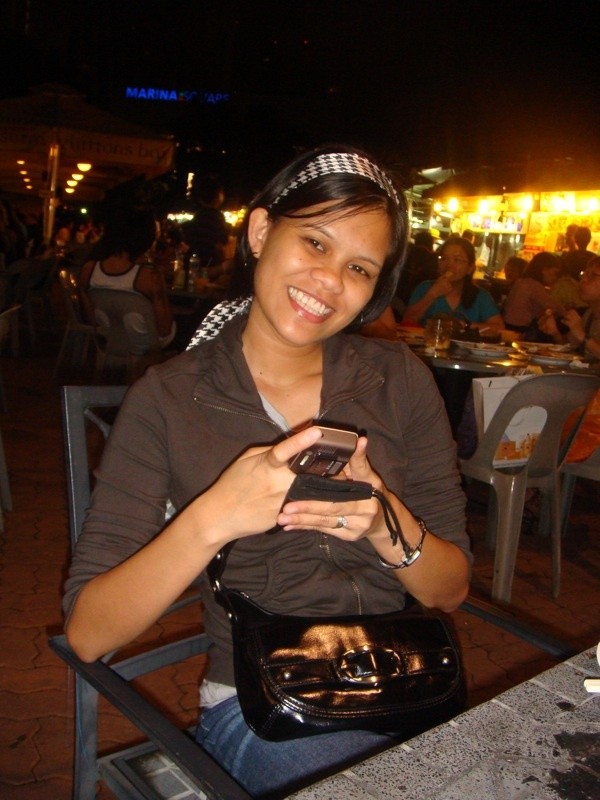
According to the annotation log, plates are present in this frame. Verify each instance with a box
[452,337,573,366]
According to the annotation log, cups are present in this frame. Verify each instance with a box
[422,317,455,357]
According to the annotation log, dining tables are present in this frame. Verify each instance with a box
[398,325,599,376]
[168,289,224,315]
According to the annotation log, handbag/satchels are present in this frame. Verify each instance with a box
[231,607,466,742]
[472,373,548,468]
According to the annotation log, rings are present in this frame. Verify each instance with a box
[335,516,345,528]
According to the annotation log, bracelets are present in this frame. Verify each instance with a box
[379,518,427,569]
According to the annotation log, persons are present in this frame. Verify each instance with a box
[58,142,473,800]
[391,233,600,505]
[0,202,234,366]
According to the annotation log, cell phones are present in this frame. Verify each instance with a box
[289,426,358,478]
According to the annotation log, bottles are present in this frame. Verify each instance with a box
[171,253,201,292]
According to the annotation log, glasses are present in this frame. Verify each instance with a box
[579,271,600,279]
[437,256,468,265]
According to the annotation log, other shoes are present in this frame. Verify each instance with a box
[522,507,538,535]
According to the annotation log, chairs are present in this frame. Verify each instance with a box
[88,284,158,384]
[48,384,576,800]
[0,302,24,533]
[49,260,94,376]
[457,371,599,604]
